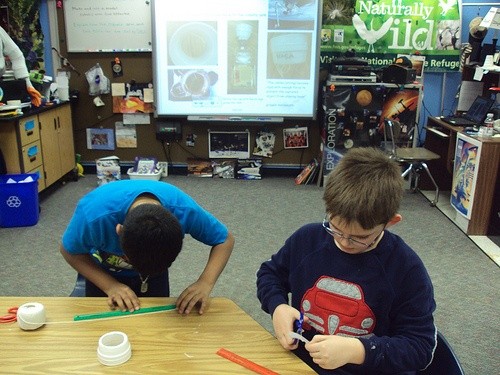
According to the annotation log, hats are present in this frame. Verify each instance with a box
[469,17,487,39]
[389,56,413,67]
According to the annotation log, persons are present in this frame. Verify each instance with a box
[0,26,42,107]
[256,147,436,375]
[58,179,236,314]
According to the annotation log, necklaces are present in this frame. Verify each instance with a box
[139,272,150,293]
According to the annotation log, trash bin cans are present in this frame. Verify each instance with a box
[0,172,41,227]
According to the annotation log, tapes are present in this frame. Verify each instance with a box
[7,100,20,105]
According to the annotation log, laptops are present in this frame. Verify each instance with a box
[441,95,495,126]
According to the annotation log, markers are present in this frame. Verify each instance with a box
[113,49,150,51]
[99,49,112,52]
[87,49,98,52]
[17,112,23,114]
[229,116,271,120]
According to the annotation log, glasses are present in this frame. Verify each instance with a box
[323,209,385,248]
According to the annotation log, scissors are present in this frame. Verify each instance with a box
[293,312,304,345]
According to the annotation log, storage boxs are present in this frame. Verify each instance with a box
[127,167,163,181]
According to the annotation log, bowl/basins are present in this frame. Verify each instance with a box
[7,100,21,105]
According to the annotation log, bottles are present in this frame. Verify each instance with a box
[491,39,498,54]
[484,114,494,138]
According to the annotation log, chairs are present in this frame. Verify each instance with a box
[384,117,441,206]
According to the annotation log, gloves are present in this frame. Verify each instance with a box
[26,80,42,107]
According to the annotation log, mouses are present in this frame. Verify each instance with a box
[465,127,478,132]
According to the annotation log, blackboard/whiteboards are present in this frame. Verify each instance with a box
[63,0,152,54]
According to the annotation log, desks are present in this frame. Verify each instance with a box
[0,296,320,375]
[421,116,500,236]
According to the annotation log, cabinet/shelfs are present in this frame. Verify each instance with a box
[0,102,76,193]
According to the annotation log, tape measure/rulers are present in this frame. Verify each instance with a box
[216,348,281,375]
[73,305,176,321]
